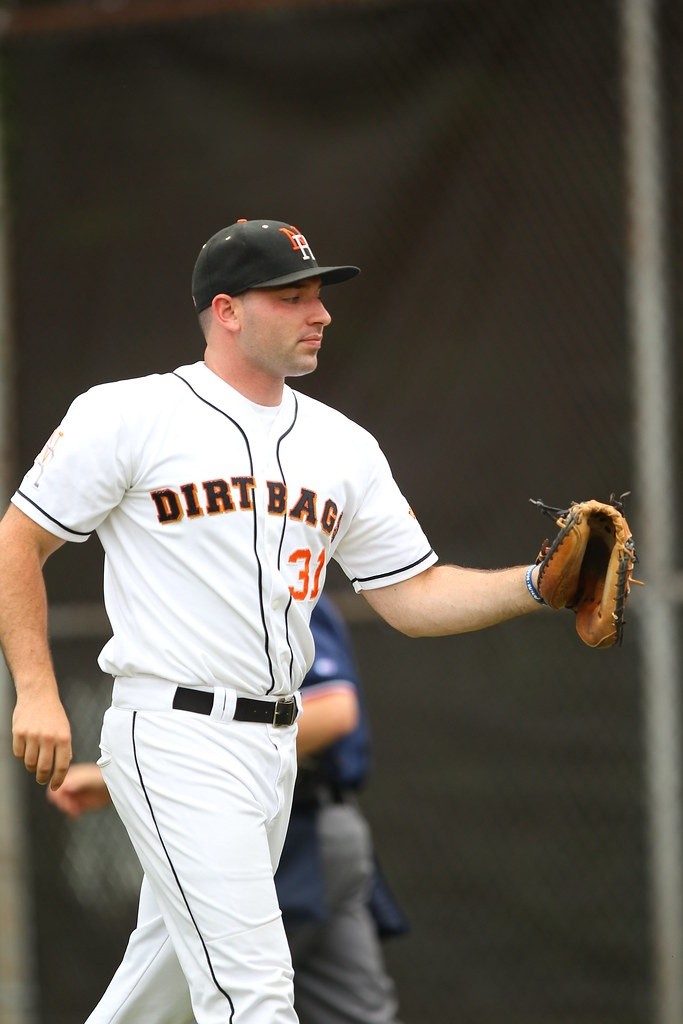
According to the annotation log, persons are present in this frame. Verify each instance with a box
[0,217,634,1023]
[48,586,409,1024]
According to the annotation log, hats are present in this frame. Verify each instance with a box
[190,219,360,314]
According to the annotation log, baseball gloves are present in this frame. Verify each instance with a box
[533,499,639,648]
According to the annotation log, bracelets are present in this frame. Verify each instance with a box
[525,564,547,605]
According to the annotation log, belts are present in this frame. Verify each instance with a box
[293,784,345,815]
[172,687,301,729]
